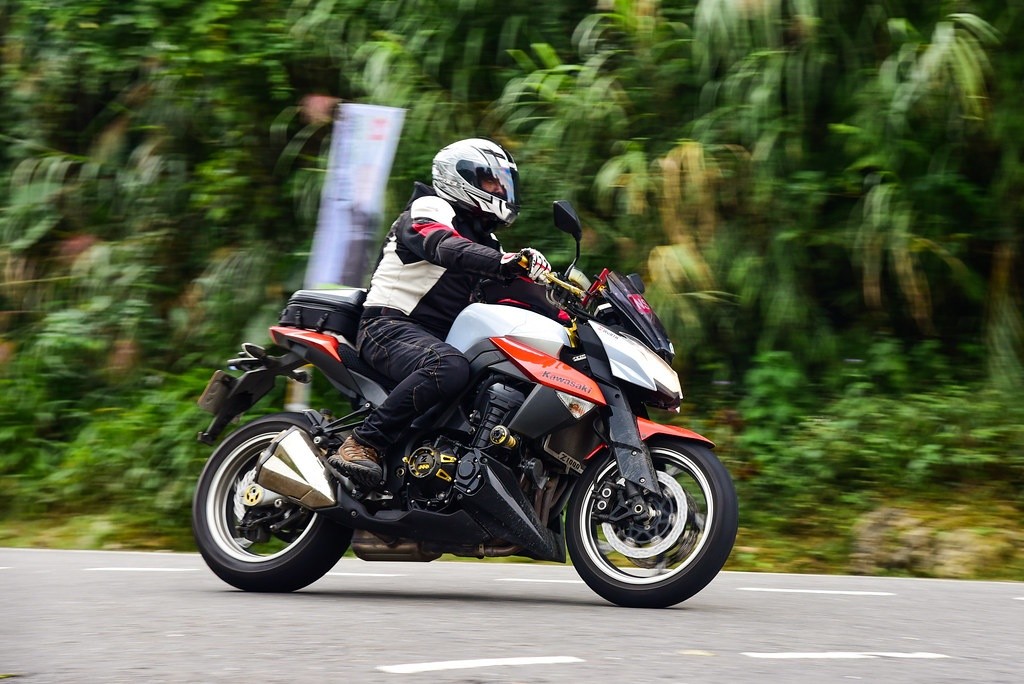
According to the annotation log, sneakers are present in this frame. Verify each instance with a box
[326,434,382,486]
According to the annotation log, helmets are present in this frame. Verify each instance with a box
[431,136,520,231]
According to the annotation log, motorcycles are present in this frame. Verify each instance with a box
[190,199,741,611]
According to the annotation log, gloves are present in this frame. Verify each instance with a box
[499,247,552,285]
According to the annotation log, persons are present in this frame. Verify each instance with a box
[328,136,551,482]
